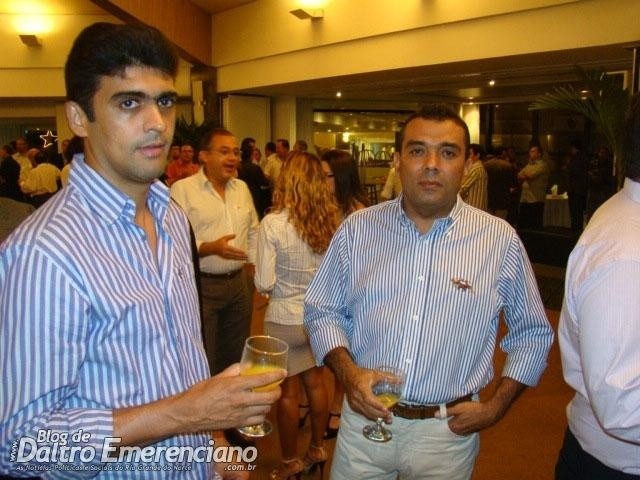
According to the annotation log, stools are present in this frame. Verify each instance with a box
[365,183,377,203]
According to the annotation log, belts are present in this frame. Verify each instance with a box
[364,394,484,418]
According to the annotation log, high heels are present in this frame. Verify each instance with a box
[324,411,340,439]
[303,446,328,478]
[297,402,308,426]
[268,459,303,476]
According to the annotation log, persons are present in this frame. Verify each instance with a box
[167,139,200,188]
[500,141,517,164]
[19,153,61,208]
[9,134,85,188]
[171,144,181,166]
[379,165,402,203]
[240,134,308,166]
[484,141,518,220]
[587,147,612,222]
[558,143,586,251]
[253,150,345,480]
[303,107,554,480]
[482,147,494,161]
[171,127,260,377]
[265,140,291,188]
[554,94,639,480]
[517,144,550,256]
[318,149,365,221]
[0,145,20,201]
[457,144,488,215]
[1,21,289,476]
[238,144,269,218]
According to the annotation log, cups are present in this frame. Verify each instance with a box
[363,365,407,442]
[237,336,288,438]
